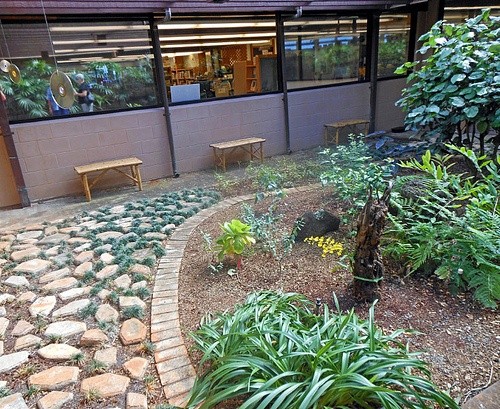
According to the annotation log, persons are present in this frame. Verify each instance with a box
[48,85,71,116]
[74,73,95,113]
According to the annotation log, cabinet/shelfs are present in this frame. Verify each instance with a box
[171,68,193,87]
[246,64,257,92]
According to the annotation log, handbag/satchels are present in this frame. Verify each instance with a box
[88,93,94,101]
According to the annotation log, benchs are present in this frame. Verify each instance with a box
[74,155,143,202]
[322,117,370,148]
[209,136,267,171]
